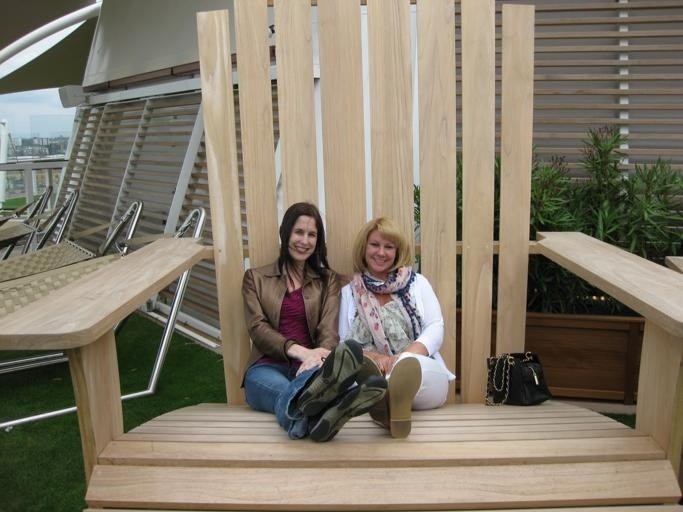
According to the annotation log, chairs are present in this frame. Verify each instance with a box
[296,339,422,442]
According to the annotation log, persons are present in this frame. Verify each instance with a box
[338,218,454,439]
[242,205,388,440]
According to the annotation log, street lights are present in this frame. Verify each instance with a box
[484,351,553,407]
